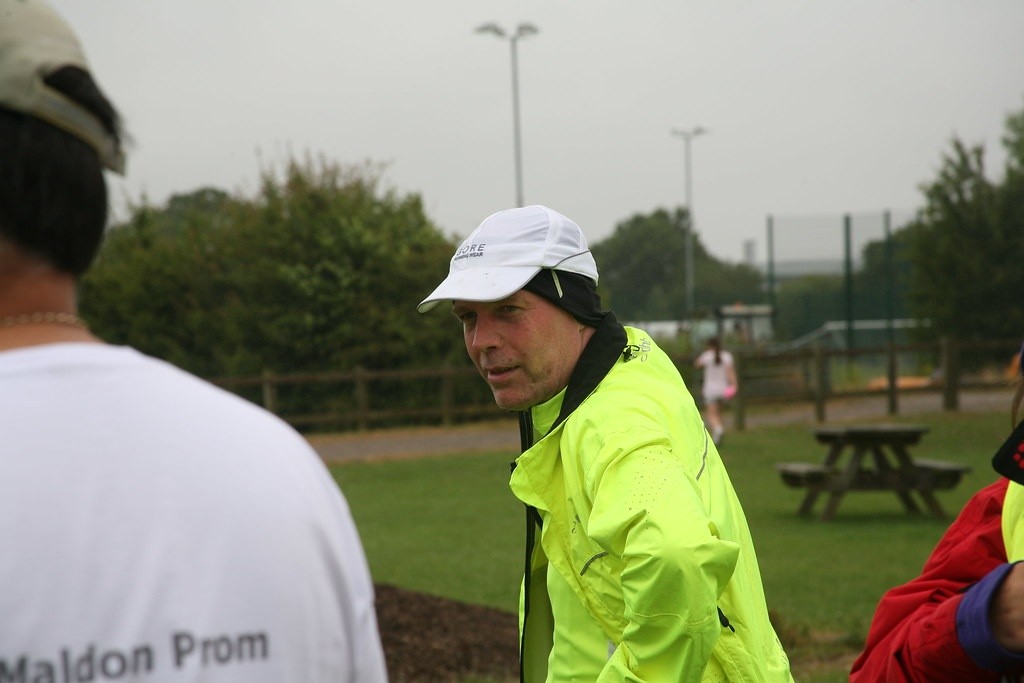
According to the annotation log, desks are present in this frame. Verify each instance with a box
[799,423,946,522]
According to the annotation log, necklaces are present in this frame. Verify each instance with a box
[0,313,86,327]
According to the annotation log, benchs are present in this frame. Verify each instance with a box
[778,461,968,492]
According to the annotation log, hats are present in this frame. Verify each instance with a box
[0,0,128,173]
[416,204,598,313]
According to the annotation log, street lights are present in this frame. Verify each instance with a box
[667,126,708,356]
[473,22,539,208]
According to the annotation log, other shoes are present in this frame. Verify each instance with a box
[713,427,724,447]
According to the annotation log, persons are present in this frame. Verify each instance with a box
[695,337,738,445]
[1,0,388,683]
[849,351,1024,683]
[417,204,793,683]
